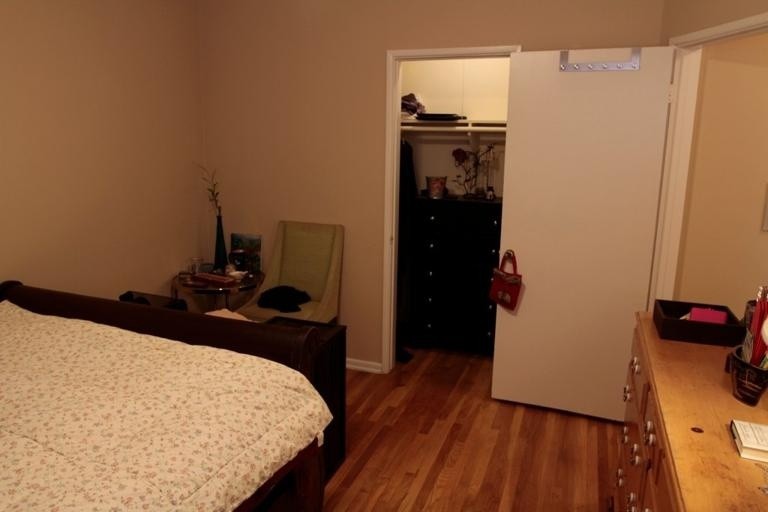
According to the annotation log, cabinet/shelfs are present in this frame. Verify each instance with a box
[119,289,188,314]
[395,193,502,359]
[612,307,766,511]
[266,315,349,488]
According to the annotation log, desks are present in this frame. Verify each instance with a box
[175,269,265,313]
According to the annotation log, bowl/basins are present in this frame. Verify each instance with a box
[228,271,248,283]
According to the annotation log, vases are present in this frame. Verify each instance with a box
[729,344,766,407]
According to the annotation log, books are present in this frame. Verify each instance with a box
[731,419,768,462]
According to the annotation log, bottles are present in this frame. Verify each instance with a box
[744,299,758,336]
[486,186,494,200]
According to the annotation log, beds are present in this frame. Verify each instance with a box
[0,274,334,511]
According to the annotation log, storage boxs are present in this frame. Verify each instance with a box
[652,298,750,348]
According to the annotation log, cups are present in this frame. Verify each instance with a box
[723,345,767,407]
[228,249,247,273]
[202,263,214,273]
[425,174,448,200]
[187,256,203,274]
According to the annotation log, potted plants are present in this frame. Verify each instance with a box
[203,169,226,270]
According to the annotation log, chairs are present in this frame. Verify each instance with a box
[234,219,345,324]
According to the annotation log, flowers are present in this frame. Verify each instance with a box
[450,146,476,194]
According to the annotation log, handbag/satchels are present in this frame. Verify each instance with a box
[489,250,522,311]
